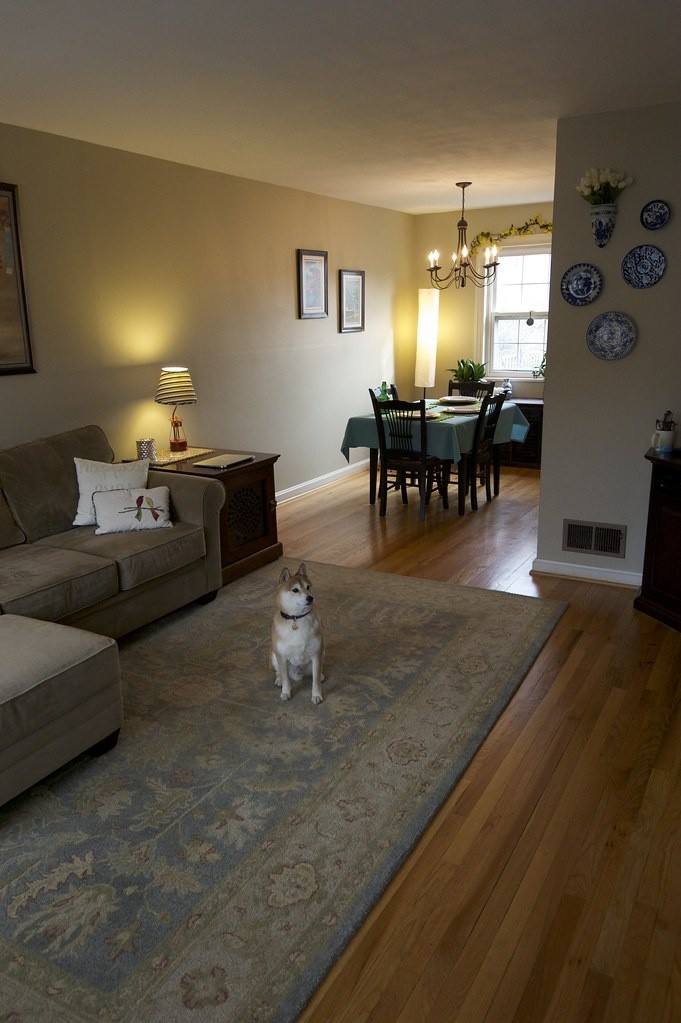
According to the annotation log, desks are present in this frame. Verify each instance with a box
[474,396,544,470]
[632,445,681,632]
[340,399,530,511]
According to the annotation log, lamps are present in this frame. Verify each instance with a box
[426,181,501,291]
[154,366,198,452]
[414,288,439,399]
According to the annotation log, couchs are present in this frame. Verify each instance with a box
[0,426,226,817]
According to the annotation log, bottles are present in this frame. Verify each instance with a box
[501,378,512,400]
[377,381,390,414]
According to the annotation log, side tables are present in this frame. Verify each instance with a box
[121,445,282,588]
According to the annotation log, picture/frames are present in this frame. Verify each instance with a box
[297,249,329,319]
[338,269,365,333]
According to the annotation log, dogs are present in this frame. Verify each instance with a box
[270,562,327,706]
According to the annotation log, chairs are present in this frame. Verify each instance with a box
[375,384,438,498]
[426,388,509,513]
[429,380,494,487]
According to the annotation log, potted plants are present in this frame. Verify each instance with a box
[443,358,490,396]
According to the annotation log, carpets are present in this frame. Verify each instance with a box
[0,556,570,1023]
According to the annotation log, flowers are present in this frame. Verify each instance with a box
[575,165,635,207]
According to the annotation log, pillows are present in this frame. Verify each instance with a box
[92,485,174,536]
[72,457,150,525]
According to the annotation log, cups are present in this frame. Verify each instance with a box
[136,438,157,462]
[651,429,676,453]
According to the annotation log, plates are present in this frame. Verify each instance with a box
[640,199,671,231]
[439,395,478,403]
[560,262,602,306]
[620,244,666,289]
[398,412,440,418]
[444,406,482,413]
[586,311,638,361]
